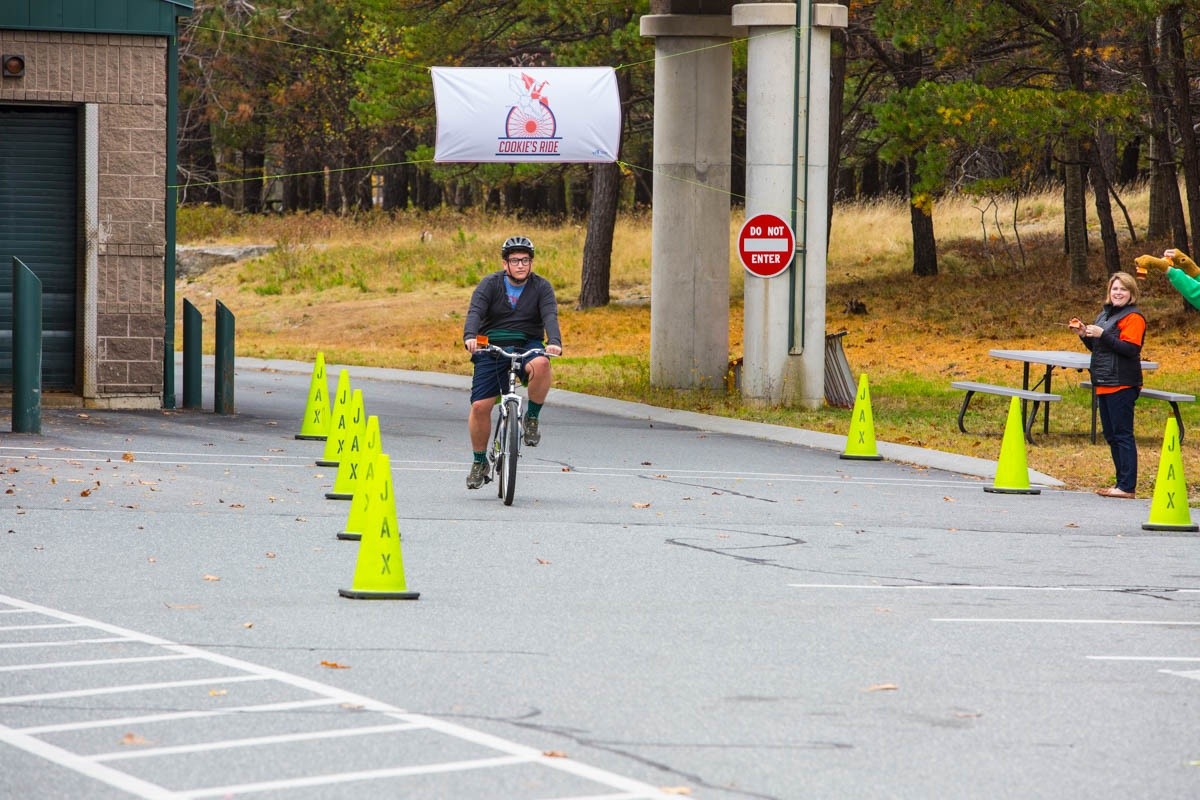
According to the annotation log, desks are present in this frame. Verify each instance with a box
[989,349,1157,443]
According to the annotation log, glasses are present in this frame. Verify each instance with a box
[508,256,534,266]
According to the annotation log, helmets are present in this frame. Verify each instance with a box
[501,237,534,259]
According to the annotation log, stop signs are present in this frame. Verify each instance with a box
[734,214,795,281]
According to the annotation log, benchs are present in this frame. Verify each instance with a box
[950,382,1195,446]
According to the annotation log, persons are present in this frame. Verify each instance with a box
[463,235,563,489]
[1133,248,1200,308]
[1069,272,1147,499]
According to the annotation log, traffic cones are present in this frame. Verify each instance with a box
[296,351,335,439]
[336,411,401,543]
[981,394,1044,495]
[837,373,886,461]
[316,373,356,467]
[322,388,369,499]
[1138,415,1200,534]
[335,451,423,603]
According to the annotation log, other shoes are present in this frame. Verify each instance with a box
[1095,483,1136,498]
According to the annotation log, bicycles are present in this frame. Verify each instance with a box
[463,340,563,510]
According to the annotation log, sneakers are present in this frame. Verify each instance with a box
[466,459,491,489]
[522,412,542,447]
